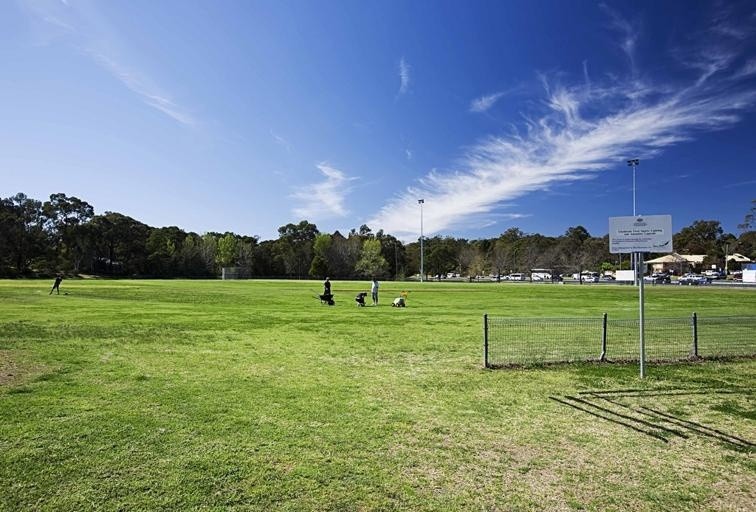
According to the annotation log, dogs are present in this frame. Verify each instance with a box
[355,291,368,298]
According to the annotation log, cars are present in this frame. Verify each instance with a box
[733,273,743,281]
[602,275,616,280]
[677,271,726,286]
[573,271,601,283]
[531,272,563,282]
[491,272,526,281]
[644,273,671,284]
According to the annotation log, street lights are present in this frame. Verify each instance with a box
[626,157,643,287]
[417,198,425,282]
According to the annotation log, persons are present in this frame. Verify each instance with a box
[371,277,378,305]
[354,293,367,308]
[48,274,63,296]
[322,276,330,295]
